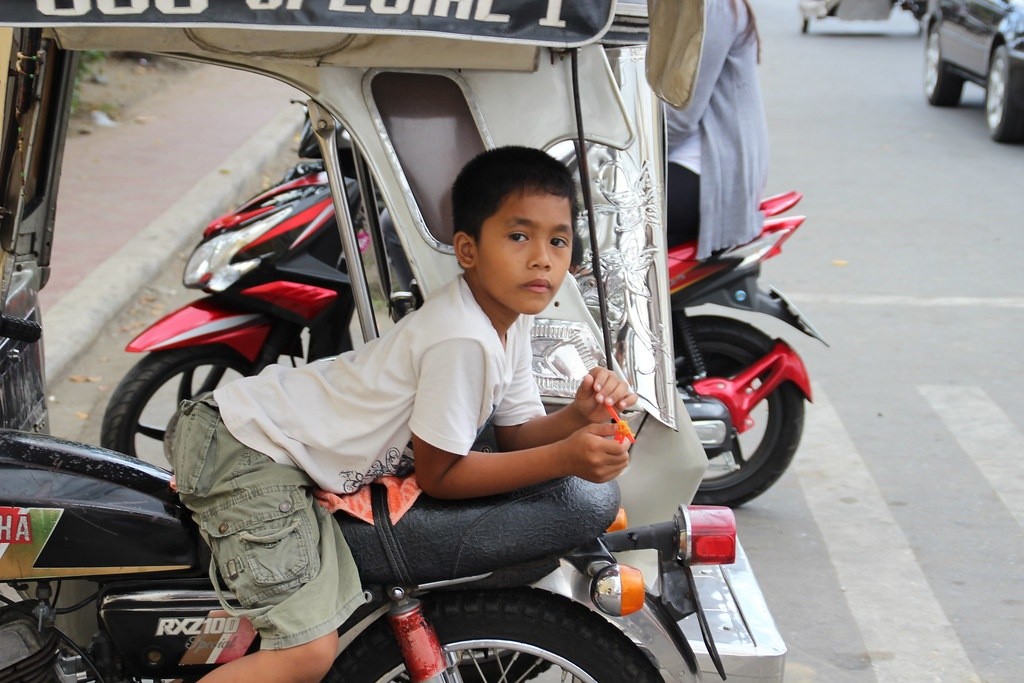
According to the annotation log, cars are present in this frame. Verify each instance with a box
[921,0,1024,146]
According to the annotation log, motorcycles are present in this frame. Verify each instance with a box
[0,0,829,683]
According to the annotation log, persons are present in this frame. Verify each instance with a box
[668,0,766,262]
[164,147,638,683]
